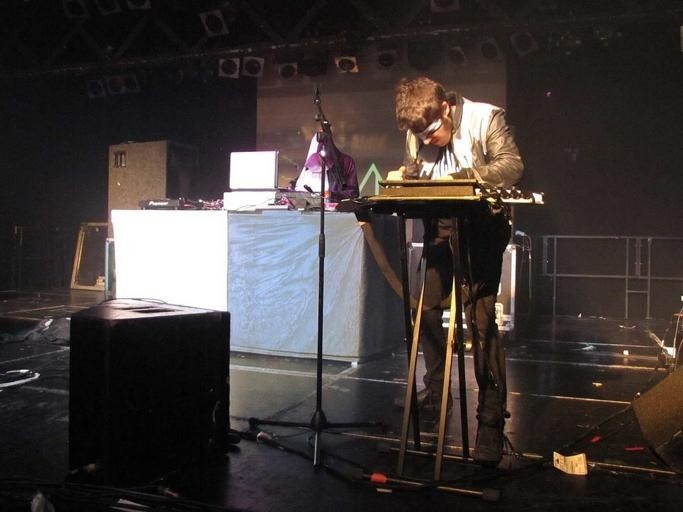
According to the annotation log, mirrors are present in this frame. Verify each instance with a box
[69,221,109,291]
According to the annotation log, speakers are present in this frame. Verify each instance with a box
[69,298,230,474]
[633,366,683,475]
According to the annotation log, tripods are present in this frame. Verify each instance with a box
[247,87,385,468]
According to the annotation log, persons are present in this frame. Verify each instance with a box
[386,78,525,467]
[288,129,360,200]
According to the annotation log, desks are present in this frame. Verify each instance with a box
[107,208,406,363]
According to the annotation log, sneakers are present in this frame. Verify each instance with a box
[392,375,456,418]
[471,418,509,466]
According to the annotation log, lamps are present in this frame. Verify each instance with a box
[376,22,604,69]
[62,1,357,100]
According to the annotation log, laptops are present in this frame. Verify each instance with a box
[230,151,289,191]
